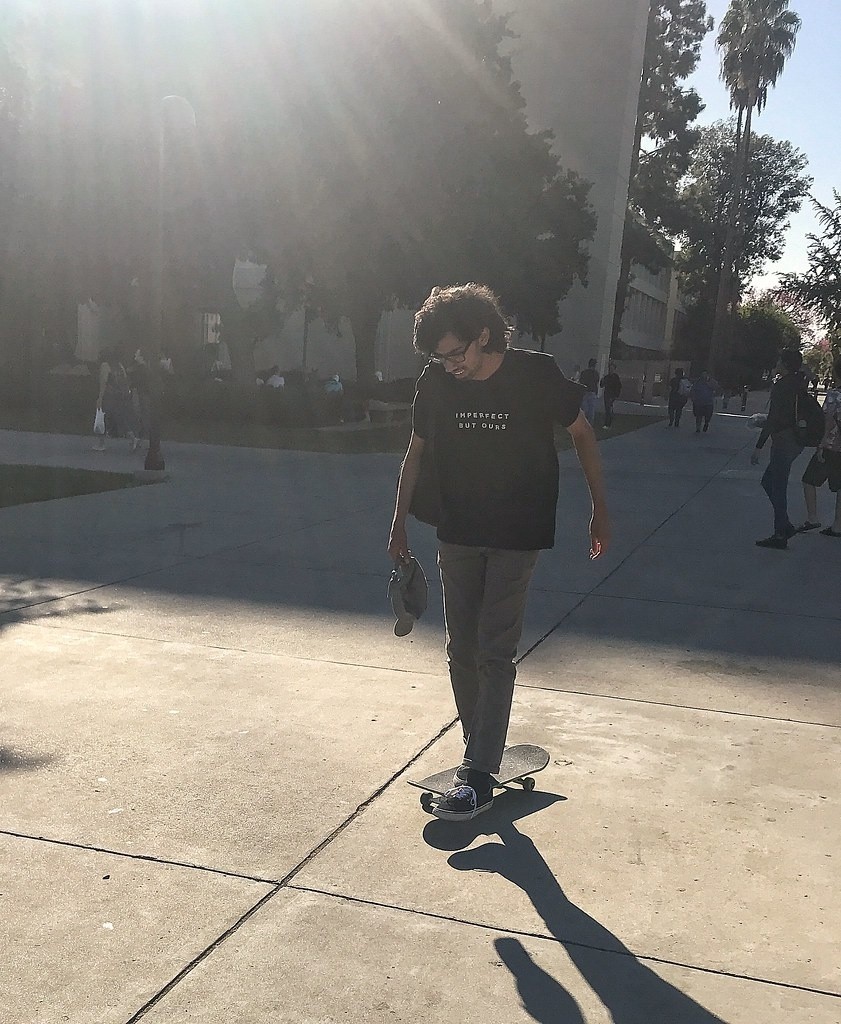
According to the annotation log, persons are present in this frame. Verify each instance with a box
[324,375,346,390]
[572,347,841,550]
[90,348,141,454]
[266,366,285,386]
[388,284,612,820]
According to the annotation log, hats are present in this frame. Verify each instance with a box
[385,557,428,638]
[779,347,804,372]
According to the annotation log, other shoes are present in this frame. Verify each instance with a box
[602,424,610,430]
[695,429,700,432]
[774,517,797,540]
[756,529,787,549]
[675,425,678,428]
[130,437,141,451]
[703,425,708,433]
[93,445,105,451]
[669,421,673,425]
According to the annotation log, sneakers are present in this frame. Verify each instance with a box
[452,764,469,788]
[432,786,494,822]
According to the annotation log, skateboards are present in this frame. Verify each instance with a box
[407,743,550,808]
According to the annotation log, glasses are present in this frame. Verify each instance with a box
[428,339,471,364]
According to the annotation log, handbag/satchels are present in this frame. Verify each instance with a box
[706,377,718,388]
[94,409,106,436]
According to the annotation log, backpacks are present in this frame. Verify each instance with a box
[787,385,828,447]
[675,377,692,395]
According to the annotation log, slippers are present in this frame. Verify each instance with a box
[798,521,821,531]
[819,527,841,538]
